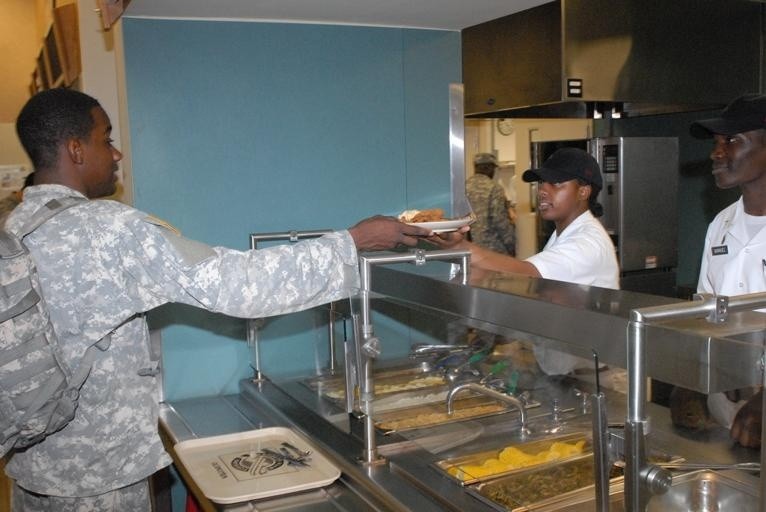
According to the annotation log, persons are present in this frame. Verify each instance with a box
[0,87,433,511]
[0,172,38,225]
[668,93,766,452]
[419,146,625,392]
[463,150,515,256]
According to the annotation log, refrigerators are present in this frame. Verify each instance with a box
[592,134,679,295]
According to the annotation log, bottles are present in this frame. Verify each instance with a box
[690,470,719,512]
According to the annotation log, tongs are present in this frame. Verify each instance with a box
[407,342,468,360]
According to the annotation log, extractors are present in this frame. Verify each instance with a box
[455,0,763,120]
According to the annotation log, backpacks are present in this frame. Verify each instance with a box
[1,195,90,461]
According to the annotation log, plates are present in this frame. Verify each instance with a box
[400,212,474,233]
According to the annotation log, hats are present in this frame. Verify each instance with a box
[690,94,766,135]
[522,148,602,188]
[474,152,500,169]
